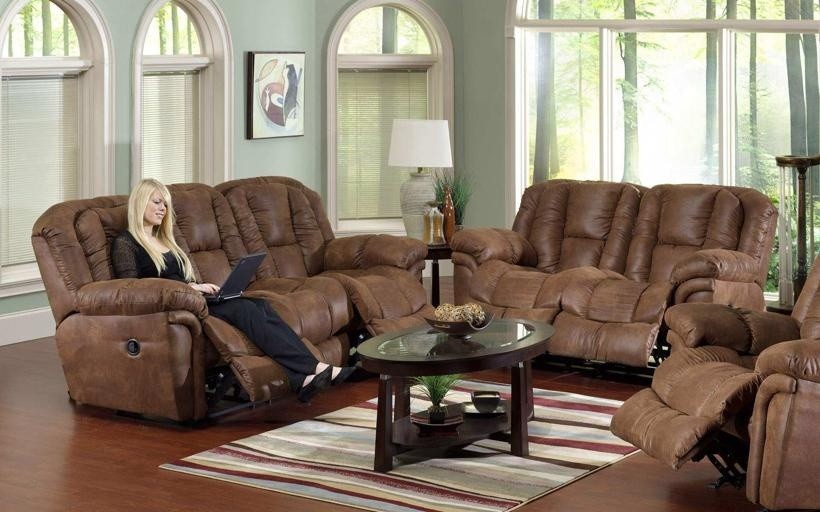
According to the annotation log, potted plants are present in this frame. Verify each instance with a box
[407,374,468,424]
[433,175,479,231]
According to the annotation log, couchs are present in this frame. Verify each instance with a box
[449,179,778,377]
[32,177,437,429]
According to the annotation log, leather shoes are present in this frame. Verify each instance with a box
[297,364,356,402]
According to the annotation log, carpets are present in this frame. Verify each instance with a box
[158,380,641,512]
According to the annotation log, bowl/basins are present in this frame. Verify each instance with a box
[471,391,501,413]
[425,336,486,357]
[423,312,498,339]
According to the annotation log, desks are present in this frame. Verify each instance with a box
[766,305,793,315]
[425,247,452,308]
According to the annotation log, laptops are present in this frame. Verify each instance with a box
[204,252,268,304]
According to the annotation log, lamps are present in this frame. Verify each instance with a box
[388,118,453,245]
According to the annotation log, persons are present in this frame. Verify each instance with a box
[110,177,357,404]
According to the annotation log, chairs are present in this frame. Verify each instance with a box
[610,251,820,511]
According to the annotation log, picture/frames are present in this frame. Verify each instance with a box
[246,51,306,140]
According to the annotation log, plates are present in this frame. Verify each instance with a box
[461,401,507,415]
